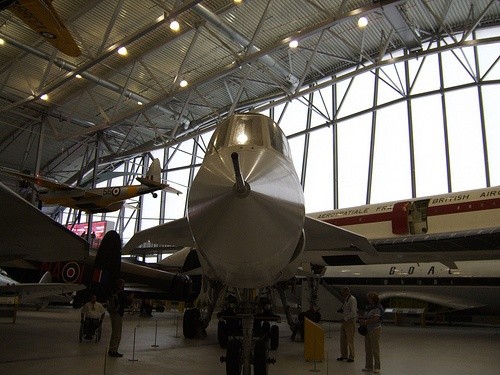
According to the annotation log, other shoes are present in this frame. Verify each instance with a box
[337,357,348,361]
[111,352,123,357]
[373,369,380,373]
[347,359,354,363]
[362,368,373,371]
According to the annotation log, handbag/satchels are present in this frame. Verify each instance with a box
[358,325,368,336]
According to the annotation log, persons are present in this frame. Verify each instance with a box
[81,294,107,340]
[107,278,134,357]
[336,289,357,364]
[356,280,382,375]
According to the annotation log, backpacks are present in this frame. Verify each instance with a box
[108,294,119,312]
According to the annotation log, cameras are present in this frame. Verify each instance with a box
[337,308,343,313]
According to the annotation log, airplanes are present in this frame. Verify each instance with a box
[323,255,500,317]
[305,184,500,259]
[0,158,183,213]
[0,179,190,319]
[121,111,378,342]
[0,269,87,323]
